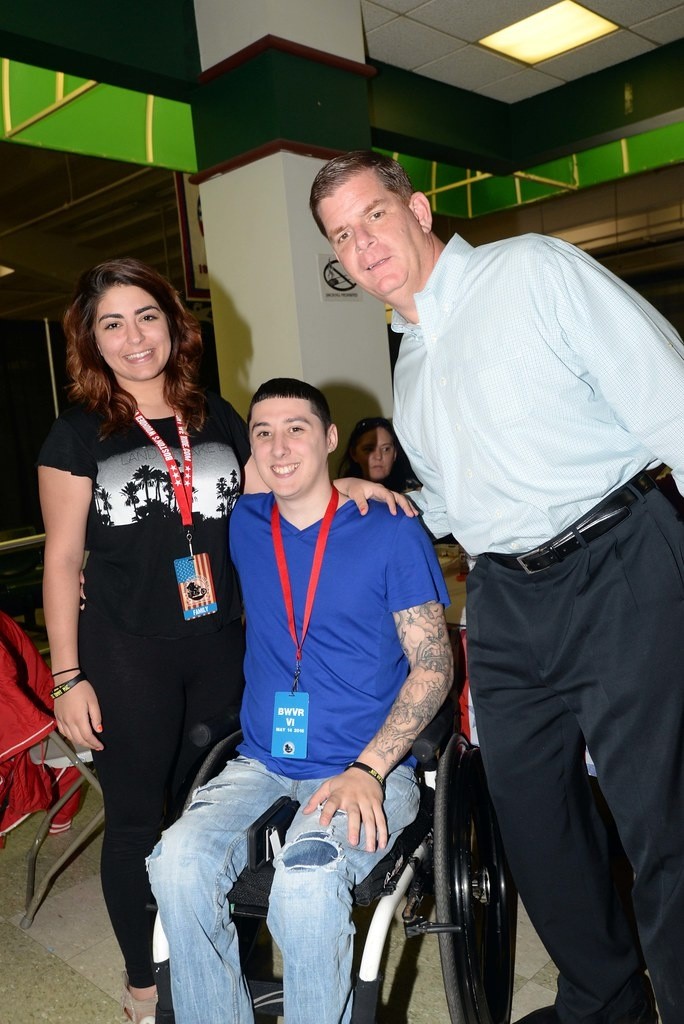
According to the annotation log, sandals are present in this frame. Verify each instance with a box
[120,970,160,1024]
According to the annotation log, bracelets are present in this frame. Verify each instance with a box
[50,667,85,700]
[344,762,385,791]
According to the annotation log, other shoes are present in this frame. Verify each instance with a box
[514,973,660,1022]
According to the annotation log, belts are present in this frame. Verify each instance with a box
[483,468,656,575]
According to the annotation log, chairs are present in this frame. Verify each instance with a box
[0,610,105,911]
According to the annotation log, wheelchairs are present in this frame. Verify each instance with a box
[147,622,518,1024]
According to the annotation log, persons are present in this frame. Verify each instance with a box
[310,150,684,1024]
[146,378,454,1024]
[37,259,419,1024]
